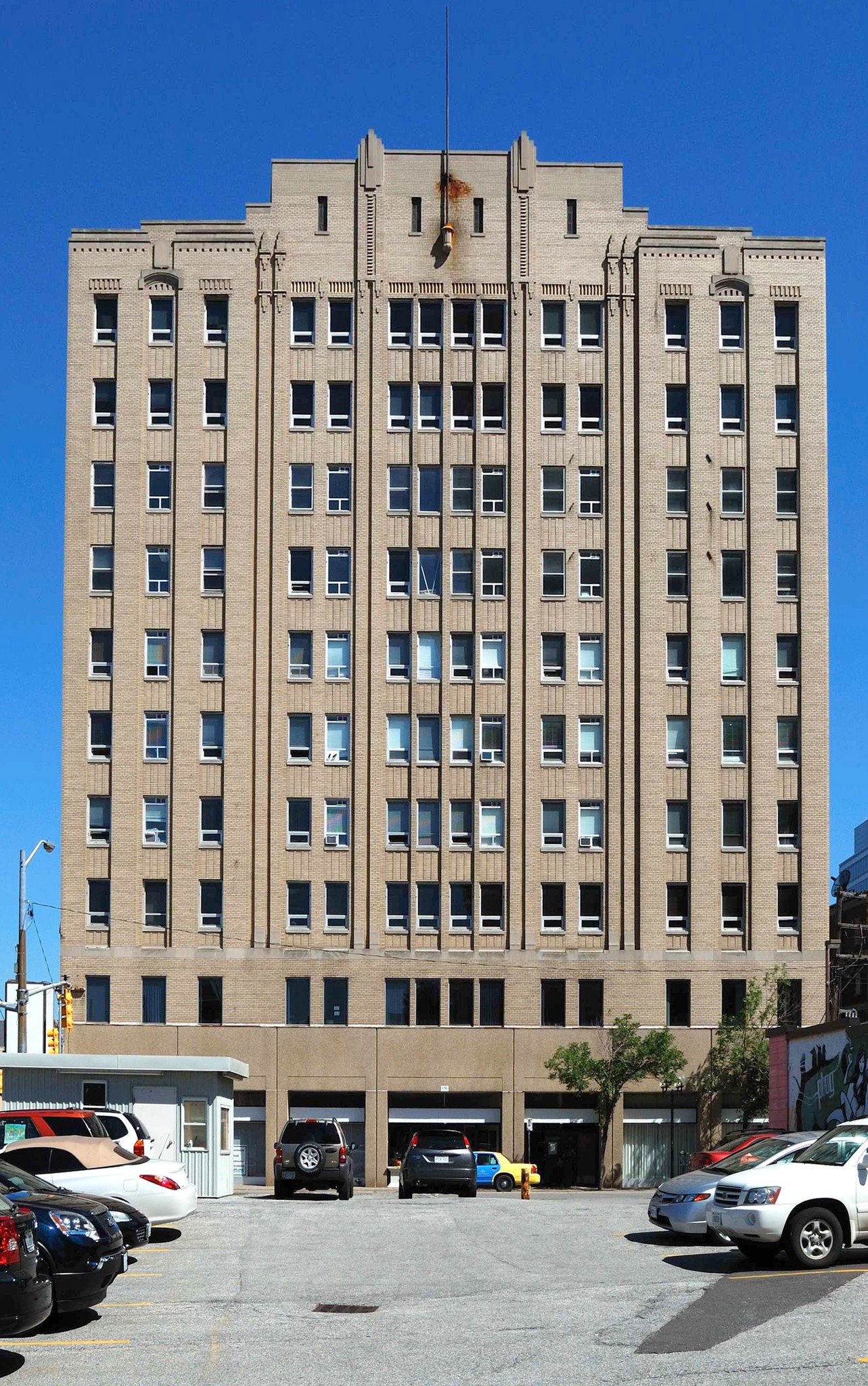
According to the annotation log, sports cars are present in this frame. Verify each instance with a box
[0,1133,197,1228]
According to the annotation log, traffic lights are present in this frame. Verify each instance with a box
[47,1027,59,1054]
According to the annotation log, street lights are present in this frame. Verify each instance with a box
[18,838,57,1055]
[825,938,842,1024]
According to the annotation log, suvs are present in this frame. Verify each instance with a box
[703,1113,868,1269]
[272,1117,358,1202]
[0,1106,155,1161]
[0,1160,129,1315]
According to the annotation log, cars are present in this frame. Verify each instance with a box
[0,1157,152,1252]
[646,1126,868,1247]
[0,1192,53,1339]
[685,1127,797,1173]
[395,1129,478,1200]
[472,1150,540,1193]
